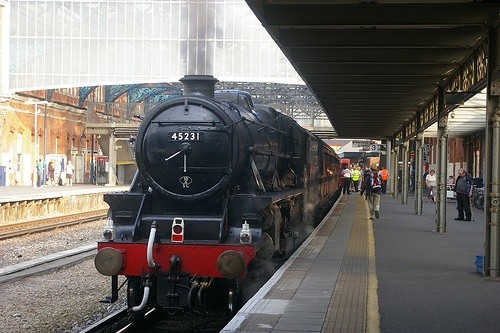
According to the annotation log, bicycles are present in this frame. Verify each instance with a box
[473,188,485,213]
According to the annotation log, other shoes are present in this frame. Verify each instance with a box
[370,217,374,220]
[455,216,464,220]
[375,210,379,219]
[464,218,471,221]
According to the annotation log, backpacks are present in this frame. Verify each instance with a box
[370,172,382,189]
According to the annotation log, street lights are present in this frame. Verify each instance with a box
[81,126,94,184]
[24,100,48,191]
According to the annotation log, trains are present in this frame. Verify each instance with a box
[90,72,350,314]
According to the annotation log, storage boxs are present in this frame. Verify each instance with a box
[474,256,484,274]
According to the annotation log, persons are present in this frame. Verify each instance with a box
[35,159,75,186]
[423,169,436,204]
[342,163,388,220]
[453,168,474,221]
